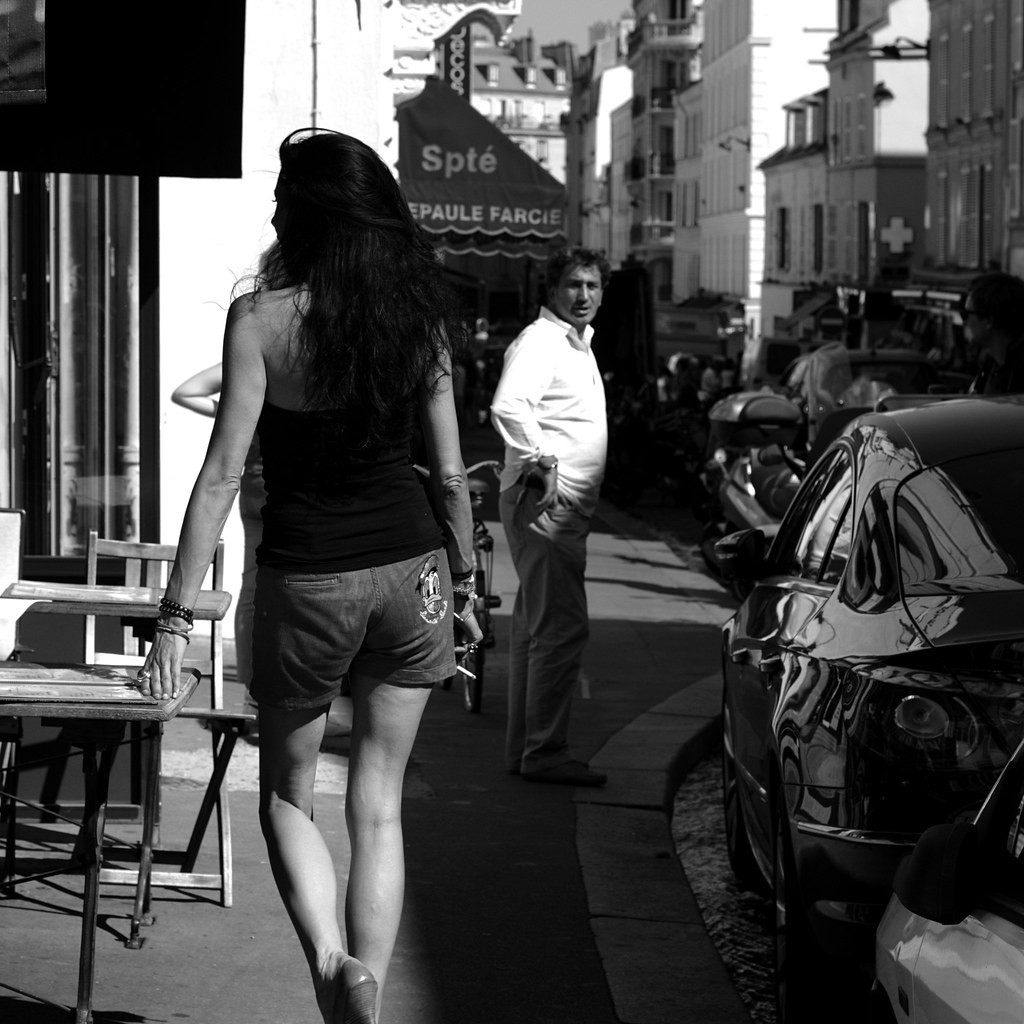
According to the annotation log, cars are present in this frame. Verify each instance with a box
[720,389,1024,1020]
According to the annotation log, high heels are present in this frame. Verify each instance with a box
[334,960,378,1024]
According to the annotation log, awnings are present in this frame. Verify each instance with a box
[396,74,568,259]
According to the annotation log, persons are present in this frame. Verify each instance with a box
[170,362,353,740]
[490,248,609,786]
[962,273,1023,397]
[135,125,480,1024]
[656,353,737,408]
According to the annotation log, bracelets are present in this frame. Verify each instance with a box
[452,582,475,595]
[158,605,194,624]
[157,616,194,631]
[156,627,191,644]
[160,598,194,616]
[453,599,474,622]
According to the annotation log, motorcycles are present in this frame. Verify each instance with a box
[698,370,989,596]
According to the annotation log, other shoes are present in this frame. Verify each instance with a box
[508,758,610,789]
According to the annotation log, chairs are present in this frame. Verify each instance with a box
[41,530,257,903]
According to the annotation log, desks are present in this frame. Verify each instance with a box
[1,659,200,1024]
